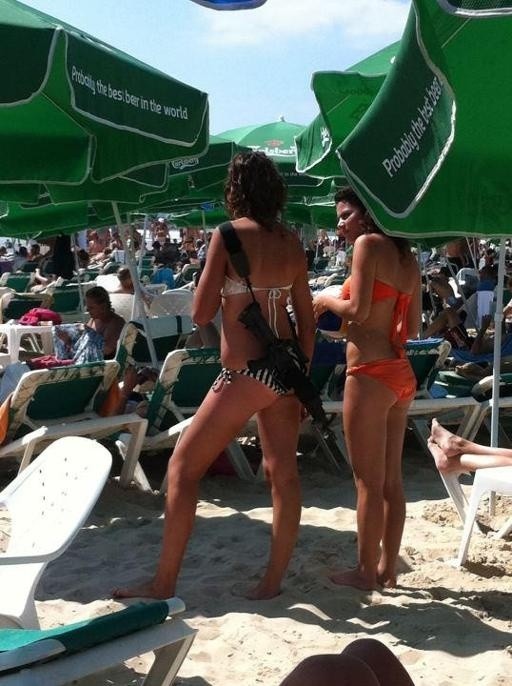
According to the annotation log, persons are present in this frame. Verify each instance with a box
[109,146,319,600]
[308,186,422,595]
[427,415,512,474]
[279,637,418,686]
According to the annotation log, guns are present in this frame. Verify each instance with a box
[238,302,347,470]
